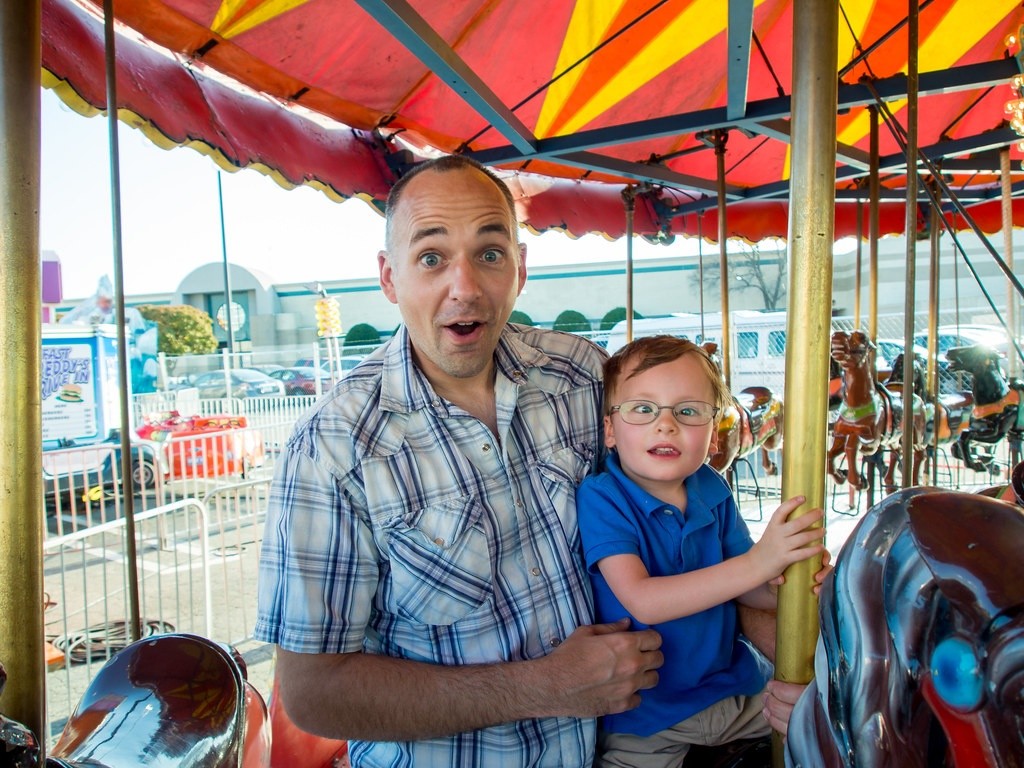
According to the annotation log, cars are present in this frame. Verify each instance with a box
[875,322,1024,420]
[294,352,370,382]
[172,367,285,412]
[268,364,337,407]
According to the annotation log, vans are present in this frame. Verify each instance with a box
[601,307,785,408]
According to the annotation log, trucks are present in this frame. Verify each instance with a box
[41,250,170,509]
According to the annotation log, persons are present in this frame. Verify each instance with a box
[575,334,833,768]
[251,155,808,768]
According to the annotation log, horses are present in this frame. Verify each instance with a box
[887,352,973,474]
[827,328,928,489]
[232,486,1024,768]
[698,342,784,477]
[944,340,1024,472]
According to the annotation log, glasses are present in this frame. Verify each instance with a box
[607,399,720,426]
